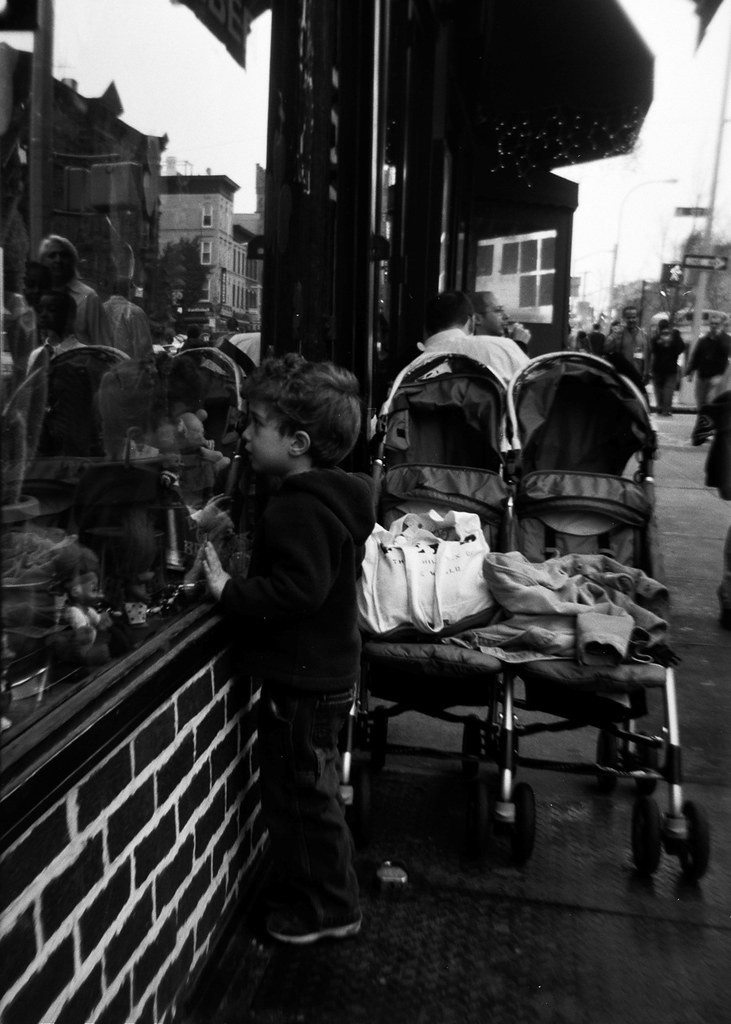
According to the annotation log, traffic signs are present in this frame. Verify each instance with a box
[683,254,728,272]
[676,207,710,218]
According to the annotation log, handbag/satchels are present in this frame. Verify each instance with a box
[352,509,495,636]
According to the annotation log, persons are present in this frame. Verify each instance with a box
[104,273,157,362]
[470,290,532,352]
[76,355,227,661]
[161,328,178,356]
[215,317,238,349]
[0,242,37,358]
[686,312,731,446]
[26,291,95,376]
[603,305,650,385]
[575,331,590,354]
[181,325,209,353]
[39,235,112,347]
[705,363,731,629]
[588,324,606,357]
[203,353,377,942]
[603,321,620,346]
[649,319,686,415]
[148,318,165,354]
[22,262,50,307]
[372,289,530,455]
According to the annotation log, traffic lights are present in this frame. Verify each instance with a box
[662,263,683,285]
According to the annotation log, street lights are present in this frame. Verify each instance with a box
[606,177,678,334]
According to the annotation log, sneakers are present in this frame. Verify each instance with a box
[266,904,362,943]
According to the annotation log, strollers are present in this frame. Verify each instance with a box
[0,344,256,722]
[334,349,713,883]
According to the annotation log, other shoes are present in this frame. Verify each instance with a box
[656,404,672,416]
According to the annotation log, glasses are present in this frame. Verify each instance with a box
[477,305,506,315]
